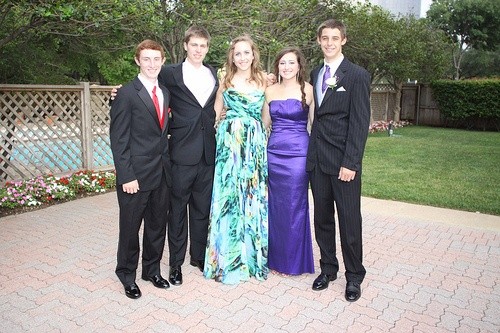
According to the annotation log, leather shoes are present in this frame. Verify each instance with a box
[168,264,183,286]
[141,273,170,289]
[345,279,361,302]
[312,271,337,291]
[123,282,141,299]
[190,257,204,272]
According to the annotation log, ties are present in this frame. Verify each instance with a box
[152,85,160,121]
[322,64,331,94]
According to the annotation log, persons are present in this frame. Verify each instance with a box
[220,47,315,281]
[266,17,371,302]
[109,39,172,299]
[202,36,279,286]
[108,25,275,287]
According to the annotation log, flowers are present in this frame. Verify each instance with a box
[216,68,227,80]
[326,75,340,91]
